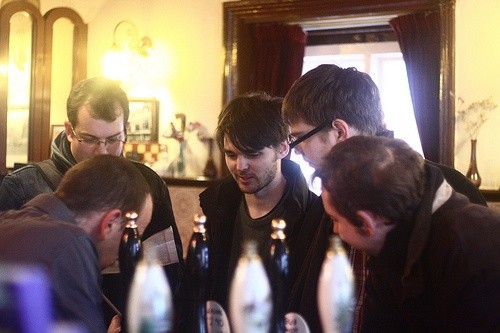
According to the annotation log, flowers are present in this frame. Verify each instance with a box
[448,92,498,140]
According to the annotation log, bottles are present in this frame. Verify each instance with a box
[204,139,217,178]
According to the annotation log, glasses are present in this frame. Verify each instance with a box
[71,126,126,145]
[286,118,336,148]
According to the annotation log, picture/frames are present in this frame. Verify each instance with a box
[123,99,158,142]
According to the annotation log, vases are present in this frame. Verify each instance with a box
[466,139,481,189]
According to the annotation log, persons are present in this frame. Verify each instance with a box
[278,64,395,333]
[311,136,500,333]
[0,154,154,333]
[0,77,184,304]
[199,92,317,333]
